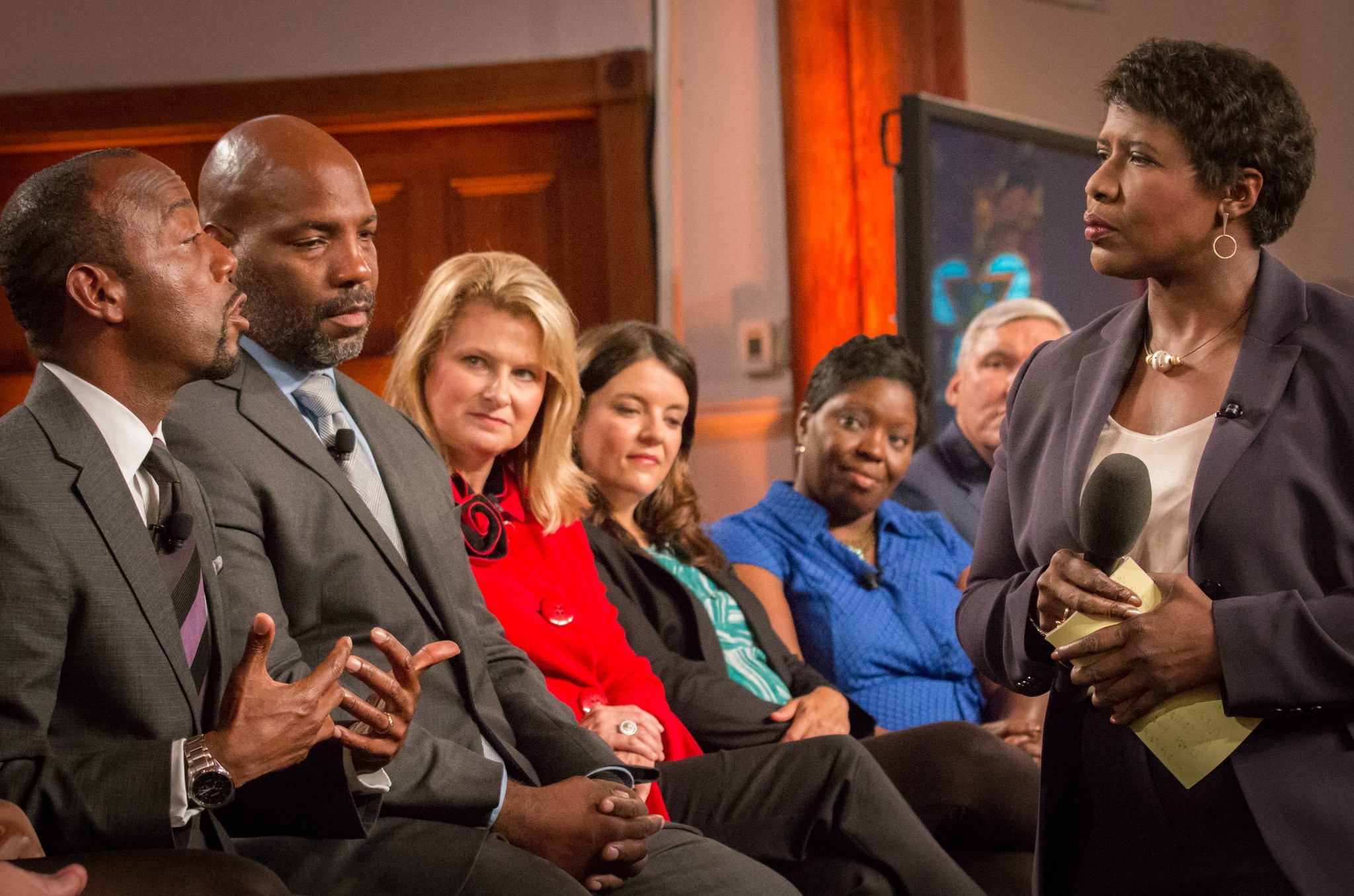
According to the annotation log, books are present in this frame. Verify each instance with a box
[1045,557,1264,789]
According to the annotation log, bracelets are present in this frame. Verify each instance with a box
[1028,612,1047,636]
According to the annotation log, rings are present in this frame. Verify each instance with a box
[374,712,392,734]
[620,720,637,735]
[1056,607,1071,627]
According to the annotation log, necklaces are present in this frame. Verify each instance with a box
[1144,298,1255,372]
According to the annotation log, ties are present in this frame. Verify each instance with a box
[142,438,212,734]
[290,374,409,572]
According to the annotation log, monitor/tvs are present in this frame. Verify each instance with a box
[895,91,1157,444]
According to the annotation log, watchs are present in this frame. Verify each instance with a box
[183,734,236,809]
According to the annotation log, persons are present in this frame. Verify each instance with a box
[953,37,1354,896]
[889,298,1073,550]
[162,114,802,896]
[700,334,1051,768]
[571,321,1040,896]
[386,252,986,896]
[0,147,461,896]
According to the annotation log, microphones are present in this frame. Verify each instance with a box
[1052,453,1153,696]
[486,494,503,512]
[335,429,354,460]
[663,534,672,548]
[170,512,194,547]
[858,570,878,590]
